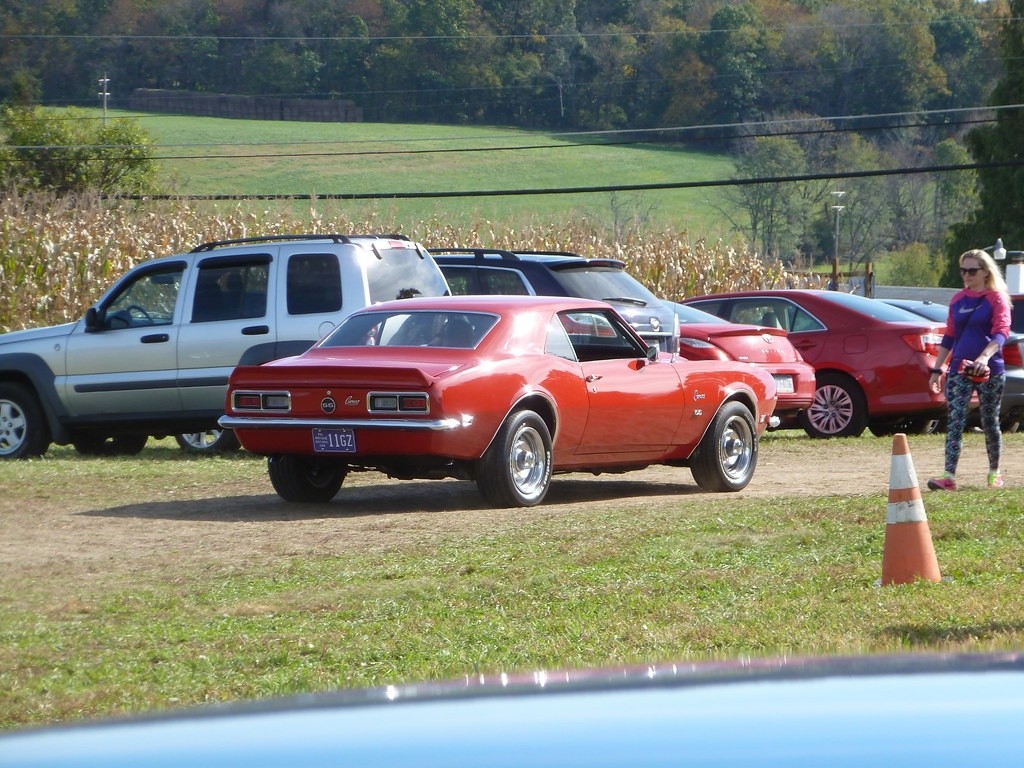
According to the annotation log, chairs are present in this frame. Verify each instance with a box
[438,319,474,347]
[761,313,781,328]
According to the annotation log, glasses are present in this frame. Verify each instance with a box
[960,266,983,276]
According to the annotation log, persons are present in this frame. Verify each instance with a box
[927,249,1013,492]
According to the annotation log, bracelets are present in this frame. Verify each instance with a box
[930,368,943,374]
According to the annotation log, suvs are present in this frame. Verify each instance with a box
[173,245,681,458]
[0,233,453,459]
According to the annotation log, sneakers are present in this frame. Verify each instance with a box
[987,470,1003,489]
[928,471,957,491]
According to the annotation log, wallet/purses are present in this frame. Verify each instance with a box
[957,359,990,382]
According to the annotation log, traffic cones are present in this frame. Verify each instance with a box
[879,433,941,585]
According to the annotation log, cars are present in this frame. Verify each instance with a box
[656,299,816,421]
[678,289,982,438]
[801,298,1024,434]
[215,292,779,506]
